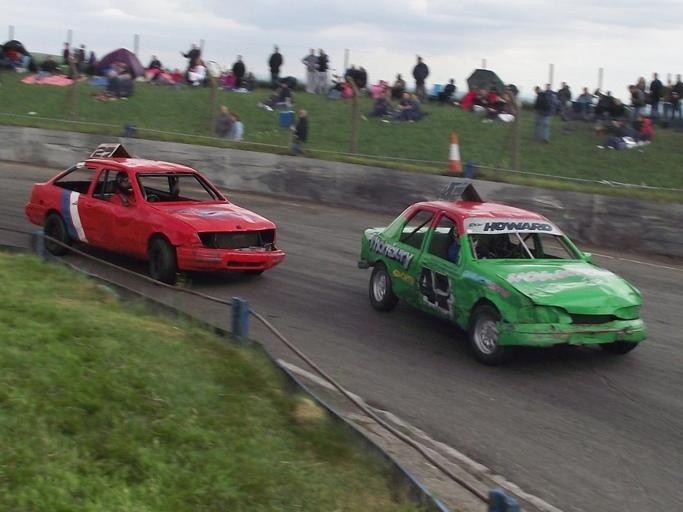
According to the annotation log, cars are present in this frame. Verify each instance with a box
[24,142,286,286]
[358,181,648,365]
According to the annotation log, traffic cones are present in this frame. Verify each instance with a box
[446,130,463,174]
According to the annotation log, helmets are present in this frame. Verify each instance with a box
[116,171,133,196]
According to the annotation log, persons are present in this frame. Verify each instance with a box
[179,43,212,86]
[534,72,683,149]
[0,39,57,75]
[100,59,135,100]
[302,47,367,99]
[360,56,428,124]
[438,79,456,101]
[144,55,175,85]
[217,106,243,141]
[64,43,95,78]
[447,225,459,263]
[110,172,133,206]
[269,47,308,154]
[462,86,518,123]
[216,54,257,92]
